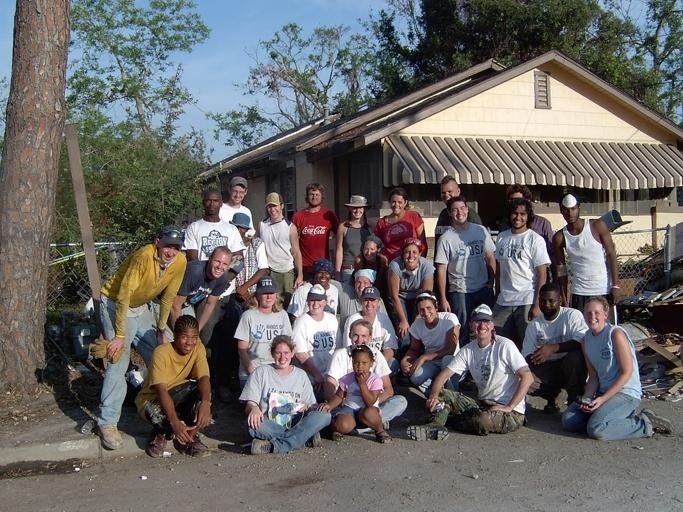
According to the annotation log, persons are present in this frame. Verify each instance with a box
[522,283,588,416]
[96,225,188,450]
[139,315,212,457]
[219,177,553,444]
[169,246,231,334]
[561,297,671,441]
[181,189,246,304]
[550,194,621,319]
[238,335,332,455]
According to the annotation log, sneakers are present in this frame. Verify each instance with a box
[642,408,674,434]
[305,433,320,448]
[97,425,123,449]
[544,389,568,414]
[376,432,392,443]
[406,423,449,441]
[251,440,271,455]
[174,436,211,457]
[148,433,166,458]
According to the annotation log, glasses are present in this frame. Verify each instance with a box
[405,238,421,245]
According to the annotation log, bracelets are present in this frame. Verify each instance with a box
[612,286,620,289]
[116,335,125,337]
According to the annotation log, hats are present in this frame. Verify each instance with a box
[362,287,380,299]
[559,193,580,213]
[230,213,250,229]
[256,276,278,295]
[471,304,493,321]
[307,284,327,301]
[230,177,247,188]
[313,259,334,273]
[344,196,368,207]
[265,193,283,207]
[157,227,185,247]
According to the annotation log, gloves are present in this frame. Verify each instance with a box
[89,339,127,364]
